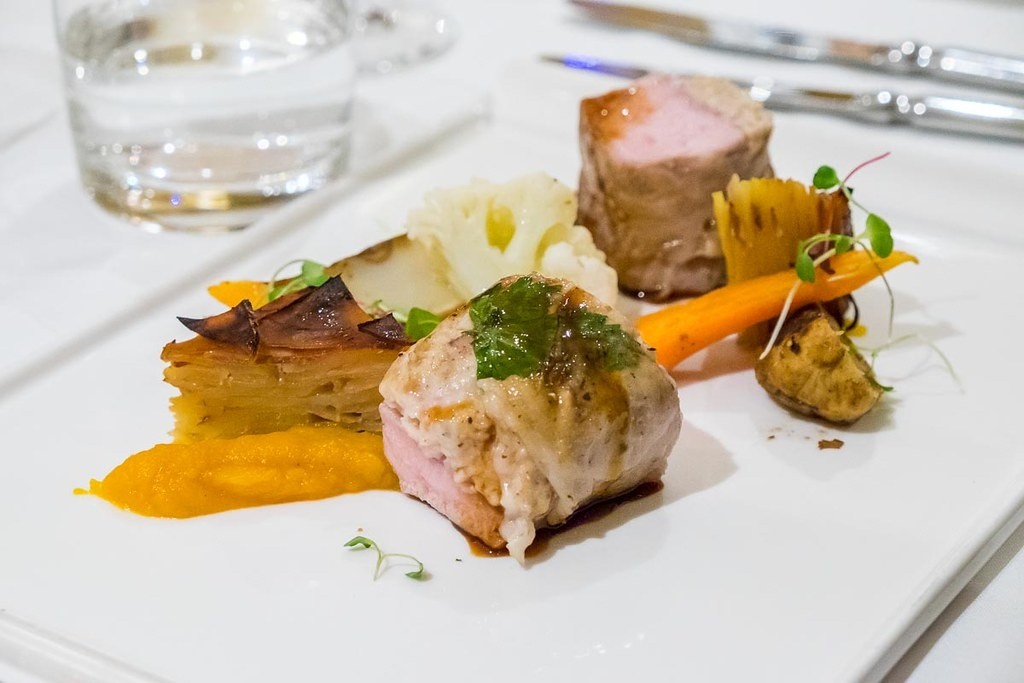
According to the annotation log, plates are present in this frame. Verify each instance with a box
[0,68,1024,683]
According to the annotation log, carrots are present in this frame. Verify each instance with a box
[636,249,919,365]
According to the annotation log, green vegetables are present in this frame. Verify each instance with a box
[757,152,894,359]
[266,256,645,381]
[344,536,424,581]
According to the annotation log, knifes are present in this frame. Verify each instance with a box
[571,1,1024,94]
[546,53,1024,143]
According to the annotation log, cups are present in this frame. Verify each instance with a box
[50,1,361,217]
